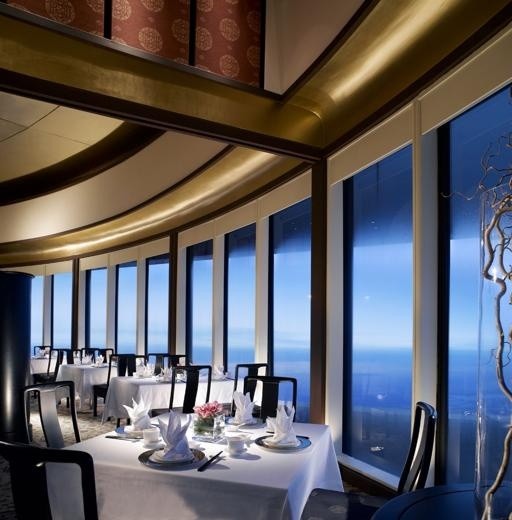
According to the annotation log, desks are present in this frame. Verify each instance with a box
[371,479,512,520]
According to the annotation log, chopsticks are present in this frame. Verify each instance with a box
[265,431,310,439]
[198,450,223,472]
[105,435,144,440]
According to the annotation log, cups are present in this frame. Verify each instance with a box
[227,437,245,450]
[143,429,159,443]
[75,361,117,367]
[132,371,232,380]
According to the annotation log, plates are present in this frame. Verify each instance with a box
[32,355,57,359]
[137,447,205,470]
[143,439,162,446]
[115,424,161,438]
[225,416,263,428]
[255,435,312,452]
[224,449,247,456]
